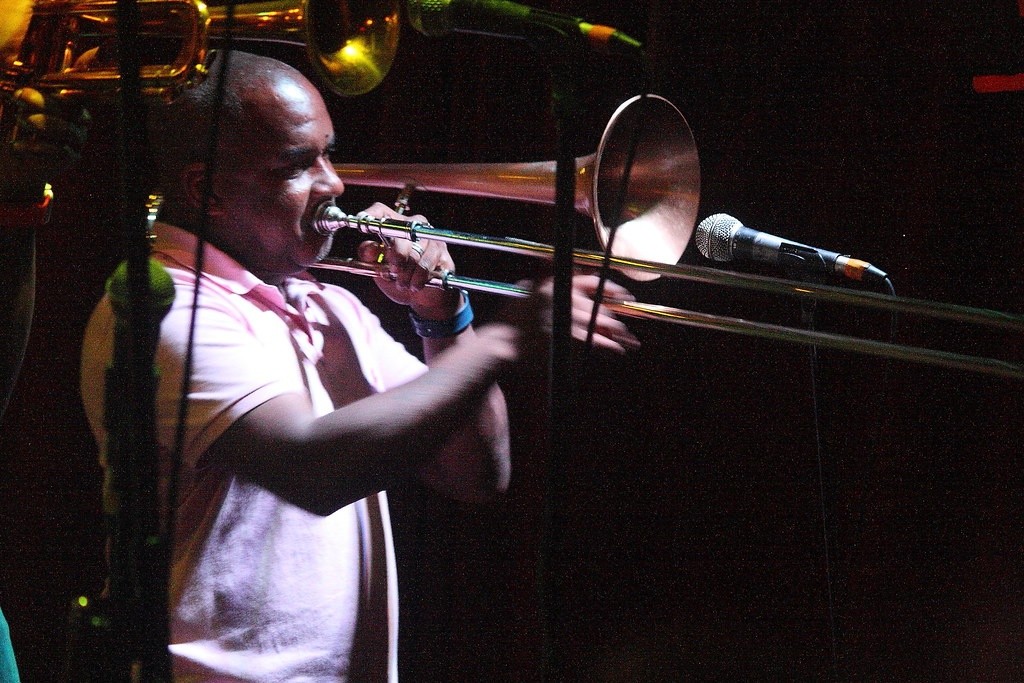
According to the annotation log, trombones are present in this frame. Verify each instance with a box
[297,91,1023,383]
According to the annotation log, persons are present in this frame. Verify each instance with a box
[81,49,639,683]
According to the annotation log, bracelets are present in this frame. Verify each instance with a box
[407,289,475,339]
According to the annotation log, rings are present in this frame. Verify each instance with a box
[411,242,424,257]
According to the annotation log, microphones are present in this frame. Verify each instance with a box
[695,211,888,286]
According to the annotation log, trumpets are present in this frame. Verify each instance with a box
[0,0,399,101]
